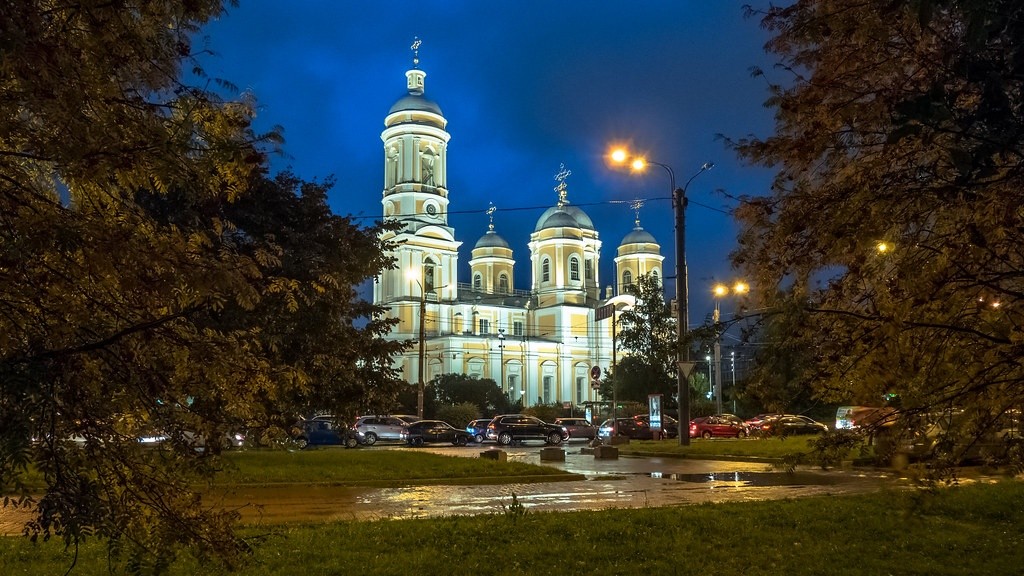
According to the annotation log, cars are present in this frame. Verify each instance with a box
[744,412,828,438]
[175,429,246,451]
[704,413,778,438]
[630,414,679,440]
[400,419,474,448]
[598,418,668,444]
[687,413,750,440]
[891,410,1024,464]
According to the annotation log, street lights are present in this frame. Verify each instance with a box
[405,267,453,421]
[712,278,752,414]
[611,148,716,446]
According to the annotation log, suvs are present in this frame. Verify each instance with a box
[466,419,493,444]
[555,418,599,442]
[310,414,360,431]
[389,415,421,423]
[293,419,367,448]
[349,415,411,445]
[486,415,565,446]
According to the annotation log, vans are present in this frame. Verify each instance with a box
[835,405,895,431]
